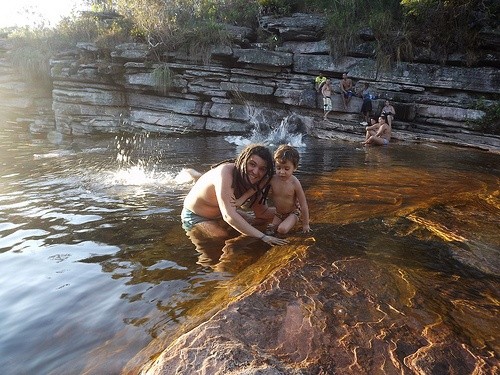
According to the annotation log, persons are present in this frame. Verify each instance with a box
[230,144,313,234]
[360,116,391,145]
[380,100,396,130]
[358,82,372,126]
[339,72,353,110]
[315,72,327,95]
[321,79,335,121]
[181,145,302,248]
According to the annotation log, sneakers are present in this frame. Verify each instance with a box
[360,122,368,125]
